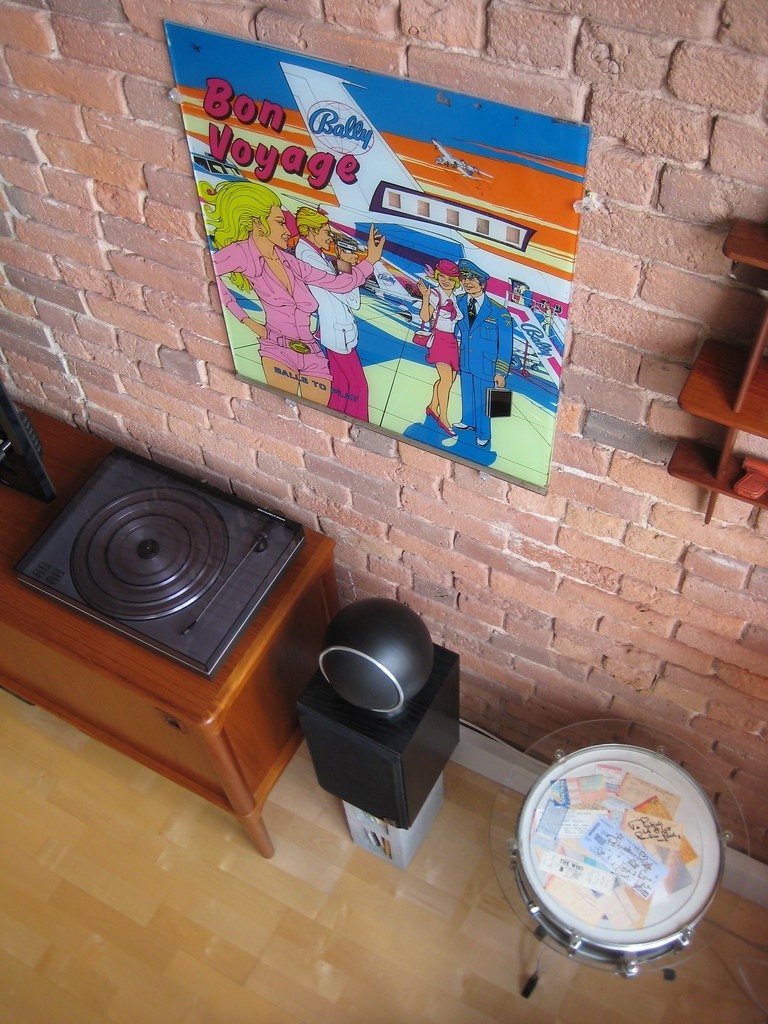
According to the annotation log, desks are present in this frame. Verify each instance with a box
[487,718,753,1000]
[0,397,341,858]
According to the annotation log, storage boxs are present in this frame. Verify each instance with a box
[341,773,443,869]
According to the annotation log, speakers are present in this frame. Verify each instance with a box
[298,641,461,830]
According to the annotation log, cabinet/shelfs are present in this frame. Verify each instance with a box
[664,218,768,525]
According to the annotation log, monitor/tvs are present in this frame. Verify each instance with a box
[0,378,57,504]
